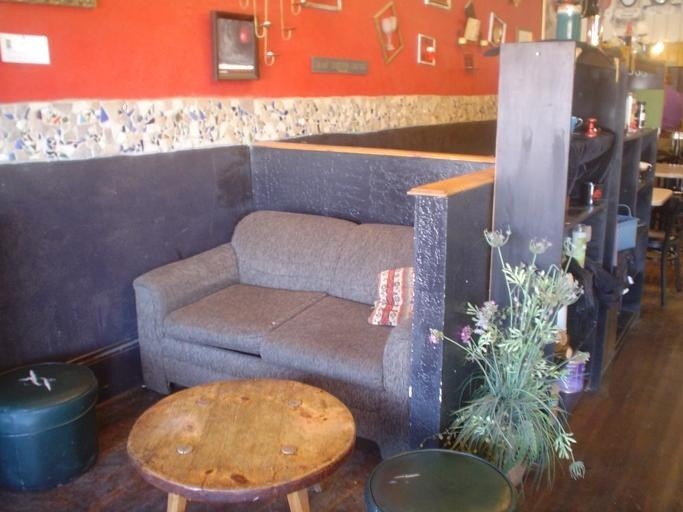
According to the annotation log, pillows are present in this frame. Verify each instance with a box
[367,264,421,333]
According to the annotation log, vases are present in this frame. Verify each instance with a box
[487,450,530,488]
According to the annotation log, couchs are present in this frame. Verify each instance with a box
[129,207,421,458]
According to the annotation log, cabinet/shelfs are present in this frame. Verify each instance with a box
[490,37,657,398]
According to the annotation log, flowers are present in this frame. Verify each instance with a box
[425,222,598,504]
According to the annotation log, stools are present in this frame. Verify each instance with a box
[364,446,520,512]
[1,361,104,494]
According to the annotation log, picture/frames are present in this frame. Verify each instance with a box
[211,8,262,83]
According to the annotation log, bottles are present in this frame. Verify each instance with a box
[625,90,639,135]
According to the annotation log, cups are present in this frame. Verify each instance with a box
[569,222,587,270]
[572,116,583,132]
